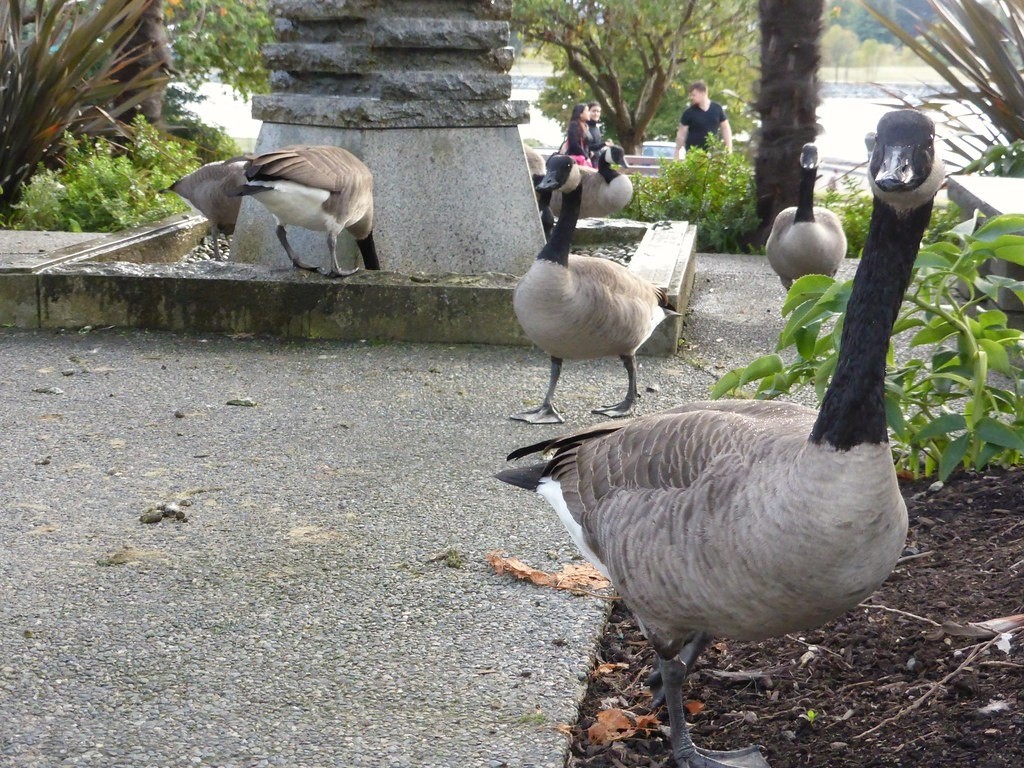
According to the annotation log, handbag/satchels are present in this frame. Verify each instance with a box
[567,154,592,166]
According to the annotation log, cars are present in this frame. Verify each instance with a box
[640,141,687,161]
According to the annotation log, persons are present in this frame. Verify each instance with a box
[564,103,594,168]
[586,101,614,169]
[672,80,733,163]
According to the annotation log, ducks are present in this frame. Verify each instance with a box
[234,145,380,278]
[156,152,255,263]
[764,141,849,294]
[523,143,555,238]
[532,144,634,226]
[495,108,948,768]
[508,154,684,424]
